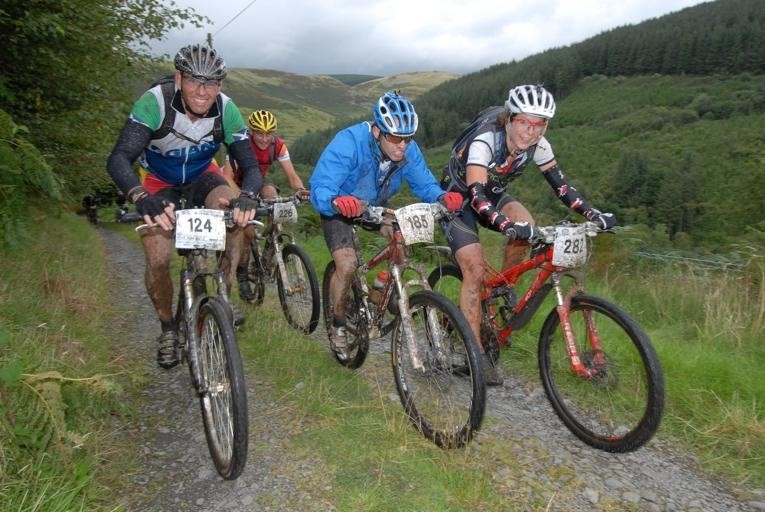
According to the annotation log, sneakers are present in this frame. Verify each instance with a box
[498,282,520,311]
[325,320,353,365]
[474,349,505,388]
[216,291,245,326]
[235,267,256,303]
[156,324,181,370]
[371,282,403,316]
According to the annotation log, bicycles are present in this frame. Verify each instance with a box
[237,186,319,335]
[133,210,271,479]
[323,199,487,447]
[421,213,664,454]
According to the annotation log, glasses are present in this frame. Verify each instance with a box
[251,130,276,140]
[179,76,226,89]
[380,133,414,145]
[509,114,547,128]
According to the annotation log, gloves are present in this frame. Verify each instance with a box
[223,190,259,212]
[583,204,617,231]
[134,191,173,218]
[438,191,464,213]
[501,219,534,241]
[293,187,311,206]
[332,194,362,218]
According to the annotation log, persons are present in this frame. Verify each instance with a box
[224,109,310,305]
[438,85,615,386]
[311,91,464,362]
[106,44,261,369]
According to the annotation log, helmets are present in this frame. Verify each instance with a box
[504,82,557,121]
[171,43,229,84]
[247,109,279,135]
[371,88,419,138]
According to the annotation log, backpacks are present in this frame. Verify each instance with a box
[151,75,225,144]
[453,106,537,171]
